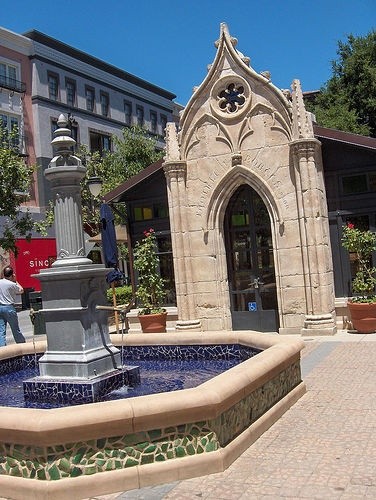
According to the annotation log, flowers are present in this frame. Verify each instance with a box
[342,223,376,303]
[133,228,168,315]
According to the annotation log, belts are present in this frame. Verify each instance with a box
[0,304,14,306]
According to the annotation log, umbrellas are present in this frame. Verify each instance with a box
[100,203,122,334]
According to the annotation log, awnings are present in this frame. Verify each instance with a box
[314,125,376,150]
[102,157,165,202]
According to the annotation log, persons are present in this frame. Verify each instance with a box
[0,266,27,347]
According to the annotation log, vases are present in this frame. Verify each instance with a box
[138,311,168,333]
[347,302,376,334]
